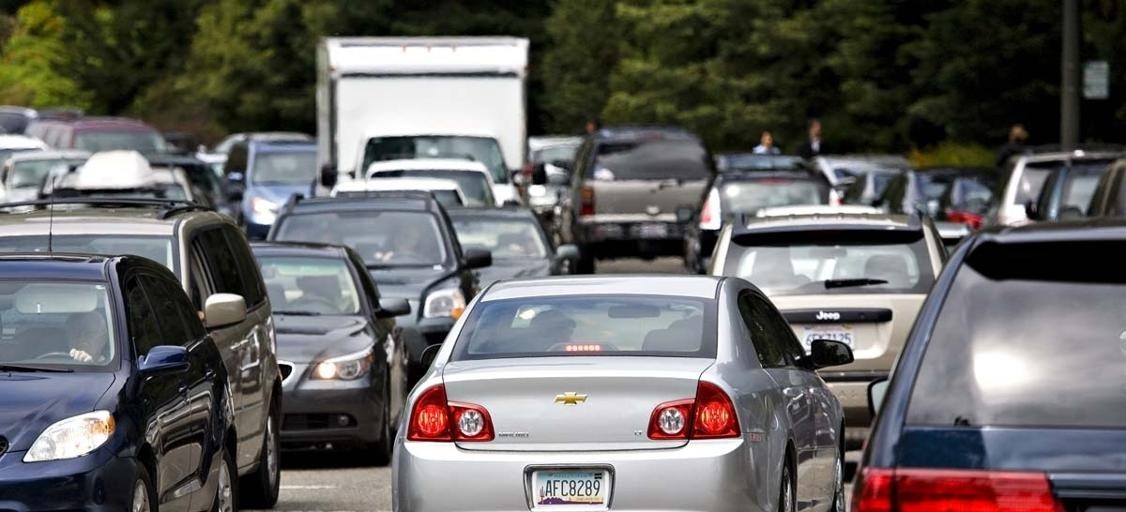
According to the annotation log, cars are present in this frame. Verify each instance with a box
[383,268,856,512]
[1,176,248,510]
[248,240,418,466]
[523,121,1126,276]
[0,103,317,237]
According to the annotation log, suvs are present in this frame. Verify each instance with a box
[846,212,1126,512]
[702,200,947,454]
[3,188,292,512]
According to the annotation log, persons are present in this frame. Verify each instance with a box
[752,131,781,154]
[585,119,600,139]
[995,123,1028,164]
[794,118,831,158]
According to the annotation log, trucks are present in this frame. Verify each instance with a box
[312,32,535,203]
